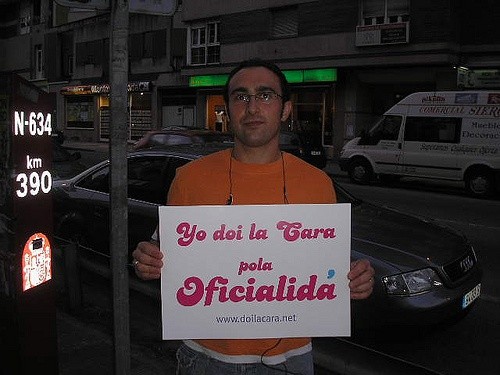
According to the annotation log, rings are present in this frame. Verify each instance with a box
[369,279,372,282]
[133,261,139,267]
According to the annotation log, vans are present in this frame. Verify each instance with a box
[337,91,500,195]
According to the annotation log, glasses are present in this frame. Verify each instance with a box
[232,91,286,104]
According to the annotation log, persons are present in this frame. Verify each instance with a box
[214,106,225,133]
[132,60,374,375]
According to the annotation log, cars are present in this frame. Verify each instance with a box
[1,146,83,224]
[45,150,483,330]
[130,127,328,170]
